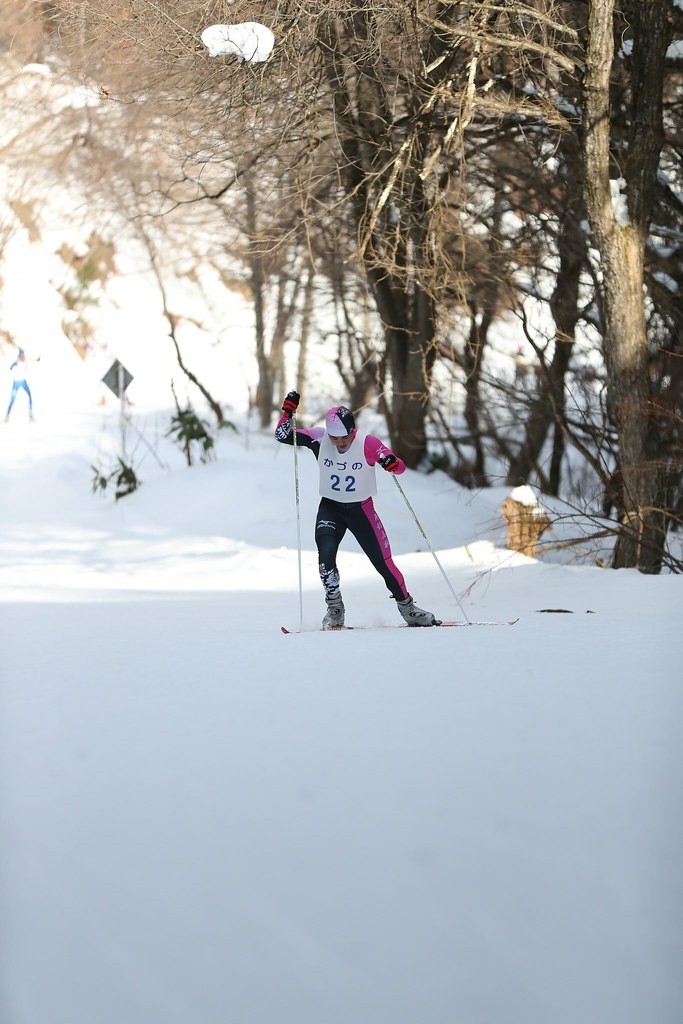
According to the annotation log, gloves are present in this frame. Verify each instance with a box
[379,454,399,472]
[282,390,300,417]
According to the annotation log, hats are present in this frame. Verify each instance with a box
[326,406,355,437]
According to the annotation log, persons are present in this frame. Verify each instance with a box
[5,348,40,421]
[275,391,435,627]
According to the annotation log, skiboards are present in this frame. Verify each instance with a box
[278,617,521,635]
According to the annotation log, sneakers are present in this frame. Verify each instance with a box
[322,592,345,627]
[390,593,435,626]
